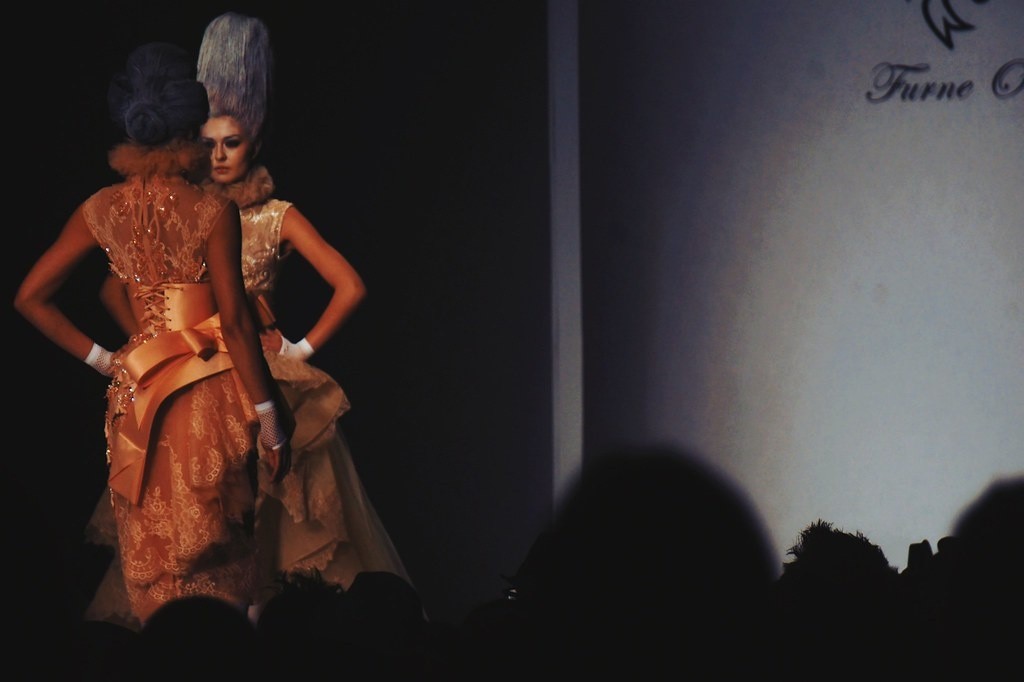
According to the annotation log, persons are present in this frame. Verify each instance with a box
[81,14,431,632]
[14,47,297,623]
[0,442,1024,682]
